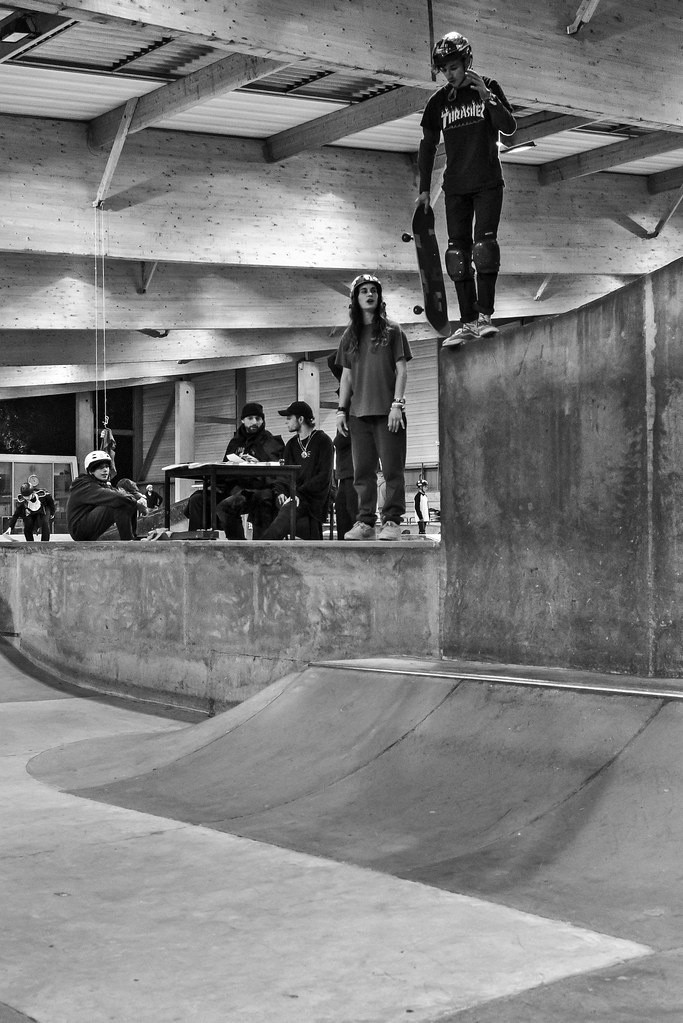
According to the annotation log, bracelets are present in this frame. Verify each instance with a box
[336,411,347,414]
[338,407,348,411]
[392,402,403,407]
[392,398,405,404]
[337,414,346,416]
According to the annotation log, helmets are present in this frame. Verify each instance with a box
[349,274,382,299]
[84,450,112,471]
[20,483,33,495]
[433,31,471,68]
[415,480,429,486]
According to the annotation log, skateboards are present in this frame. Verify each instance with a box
[3,532,19,542]
[401,201,452,338]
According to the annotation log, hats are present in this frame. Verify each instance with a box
[241,403,265,418]
[278,401,314,416]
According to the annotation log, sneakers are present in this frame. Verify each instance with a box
[379,521,402,540]
[476,318,500,336]
[443,322,479,345]
[344,521,377,540]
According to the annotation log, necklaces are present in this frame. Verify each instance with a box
[297,429,317,459]
[300,432,311,449]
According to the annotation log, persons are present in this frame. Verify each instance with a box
[216,401,335,540]
[3,483,56,541]
[145,485,163,512]
[376,459,386,524]
[100,428,117,480]
[183,402,281,540]
[415,31,517,346]
[336,274,413,541]
[327,350,359,540]
[66,450,147,541]
[414,479,430,534]
[116,478,149,517]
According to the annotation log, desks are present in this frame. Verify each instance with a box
[163,464,302,540]
